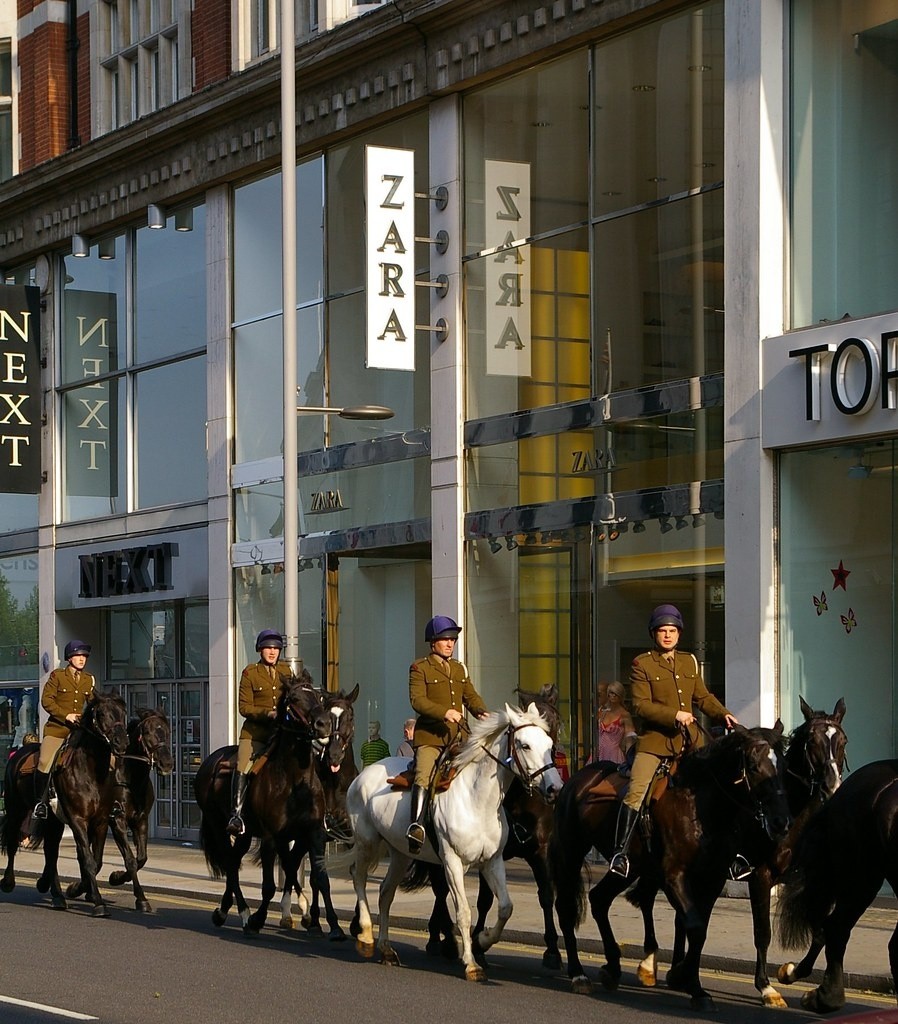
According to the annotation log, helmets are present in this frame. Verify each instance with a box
[255,630,284,651]
[63,640,92,660]
[647,605,683,634]
[424,616,463,642]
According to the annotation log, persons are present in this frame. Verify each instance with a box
[9,734,37,847]
[599,682,633,763]
[31,641,95,822]
[397,719,417,757]
[360,720,391,771]
[407,615,489,852]
[611,605,752,881]
[226,629,294,835]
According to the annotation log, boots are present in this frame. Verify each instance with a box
[227,771,248,830]
[409,783,429,854]
[610,801,640,875]
[28,768,52,817]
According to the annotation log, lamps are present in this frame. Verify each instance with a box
[71,233,94,258]
[99,237,116,259]
[175,209,195,231]
[147,203,166,229]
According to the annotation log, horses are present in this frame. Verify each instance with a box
[398,683,563,971]
[638,695,848,1011]
[774,758,898,1012]
[316,702,563,983]
[548,718,786,1016]
[280,683,362,939]
[37,700,174,912]
[191,668,348,942]
[0,687,130,918]
